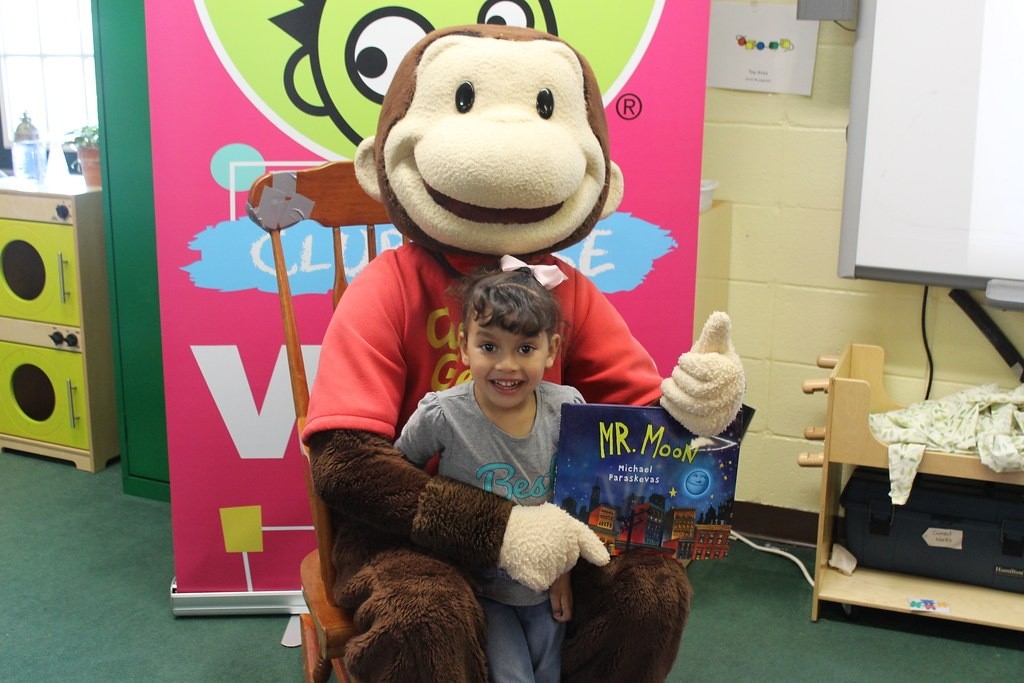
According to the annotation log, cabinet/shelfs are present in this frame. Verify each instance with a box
[796,341,1024,632]
[0,174,121,474]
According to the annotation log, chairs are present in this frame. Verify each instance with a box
[245,160,412,683]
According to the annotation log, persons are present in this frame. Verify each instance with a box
[392,253,587,681]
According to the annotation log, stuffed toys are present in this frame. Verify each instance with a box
[292,23,748,683]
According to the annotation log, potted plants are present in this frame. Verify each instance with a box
[61,124,101,187]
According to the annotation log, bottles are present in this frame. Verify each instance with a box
[11,113,46,185]
